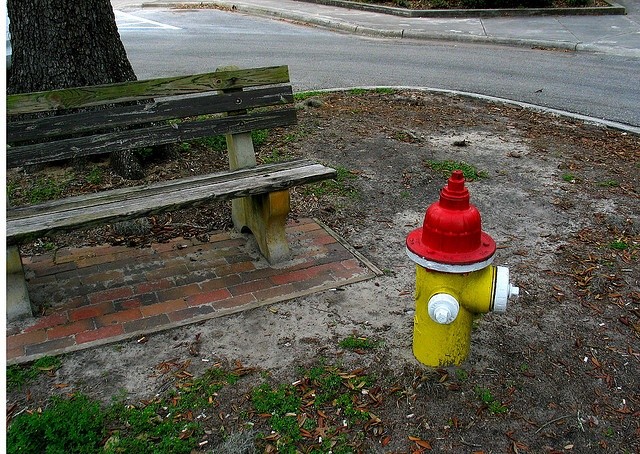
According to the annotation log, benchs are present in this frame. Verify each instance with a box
[7,65,338,324]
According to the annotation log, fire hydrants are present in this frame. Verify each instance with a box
[405,170,520,369]
